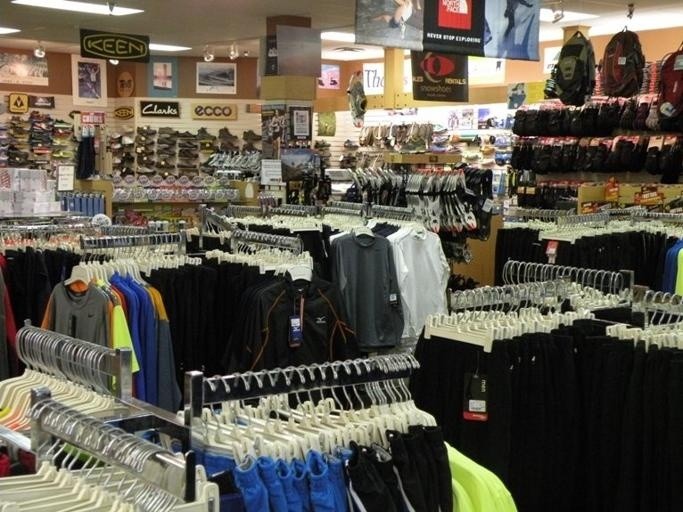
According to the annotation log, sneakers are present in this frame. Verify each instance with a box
[339,122,512,169]
[315,140,331,169]
[0,111,101,181]
[109,124,265,191]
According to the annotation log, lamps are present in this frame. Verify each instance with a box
[34,41,46,58]
[226,42,240,61]
[202,44,216,62]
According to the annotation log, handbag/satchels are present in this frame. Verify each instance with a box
[602,26,644,98]
[658,42,682,133]
[509,137,683,184]
[513,99,662,137]
[554,31,595,106]
[517,181,576,209]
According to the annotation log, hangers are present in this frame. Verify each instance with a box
[434,169,682,231]
[176,351,435,470]
[424,258,683,355]
[0,322,128,450]
[0,169,430,296]
[0,394,222,510]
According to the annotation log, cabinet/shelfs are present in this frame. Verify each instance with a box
[509,102,683,201]
[314,107,508,170]
[1,100,266,194]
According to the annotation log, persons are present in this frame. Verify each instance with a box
[266,109,284,160]
[85,63,99,97]
